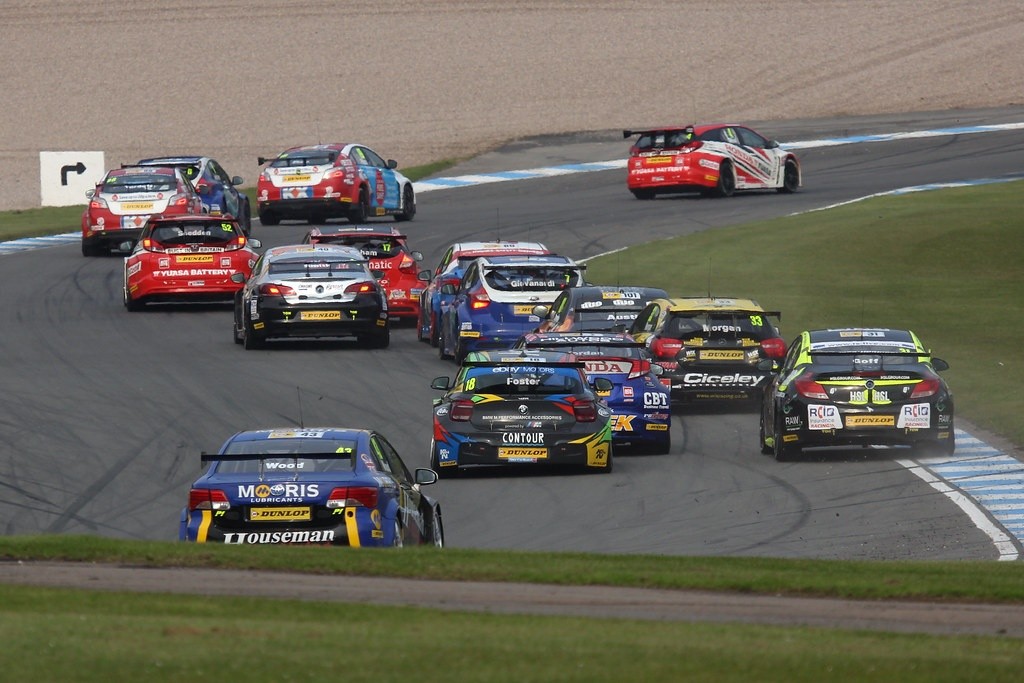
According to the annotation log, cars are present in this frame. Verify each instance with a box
[257,141,417,227]
[80,168,203,257]
[759,327,957,463]
[415,239,549,343]
[177,428,446,546]
[134,160,252,241]
[232,243,391,353]
[429,346,613,477]
[635,300,788,410]
[308,225,432,324]
[431,251,589,367]
[621,124,803,199]
[530,285,668,333]
[510,334,673,457]
[122,213,263,312]
